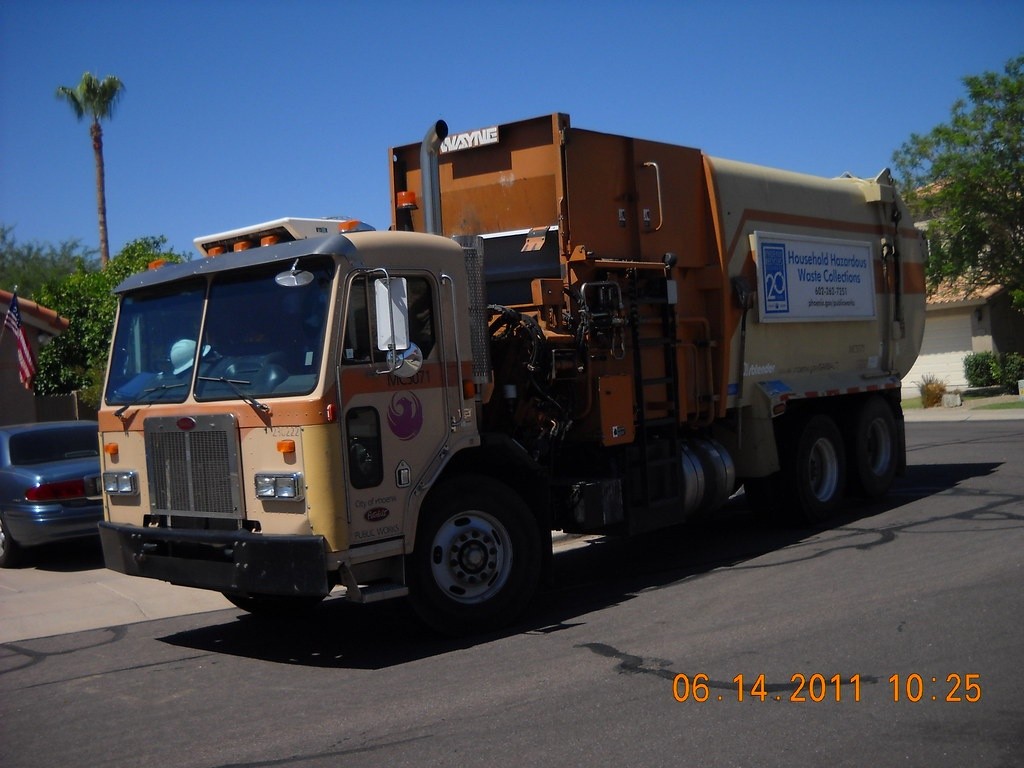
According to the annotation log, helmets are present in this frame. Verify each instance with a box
[170,340,211,375]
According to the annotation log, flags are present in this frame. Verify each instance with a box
[5,294,38,389]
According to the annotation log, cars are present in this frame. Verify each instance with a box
[1,422,104,570]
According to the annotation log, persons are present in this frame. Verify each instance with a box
[408,282,435,360]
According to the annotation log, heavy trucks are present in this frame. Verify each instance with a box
[96,111,929,635]
